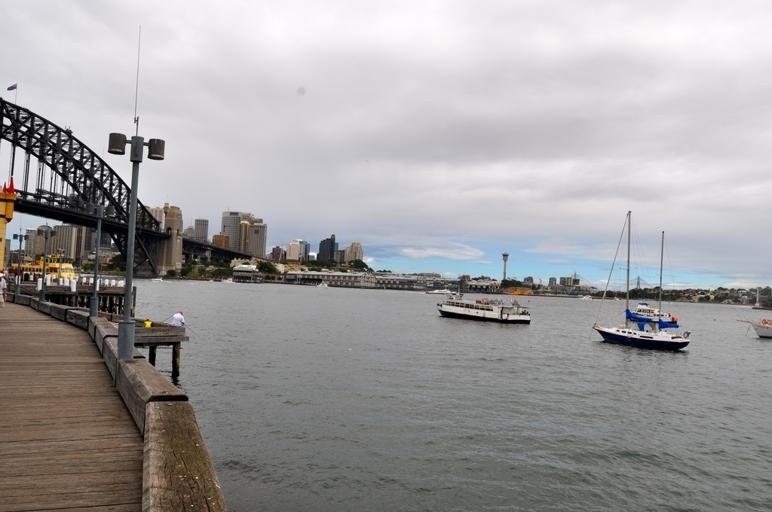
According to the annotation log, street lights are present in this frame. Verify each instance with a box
[34,221,57,300]
[107,115,167,363]
[12,226,30,284]
[81,200,115,318]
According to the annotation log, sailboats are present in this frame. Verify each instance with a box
[589,210,693,352]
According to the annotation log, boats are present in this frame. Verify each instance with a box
[317,281,328,288]
[735,318,771,338]
[626,298,682,329]
[435,295,531,326]
[11,249,77,282]
[425,289,457,295]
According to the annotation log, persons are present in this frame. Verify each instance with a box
[144,319,152,327]
[172,308,185,326]
[30,268,33,275]
[0,266,21,307]
